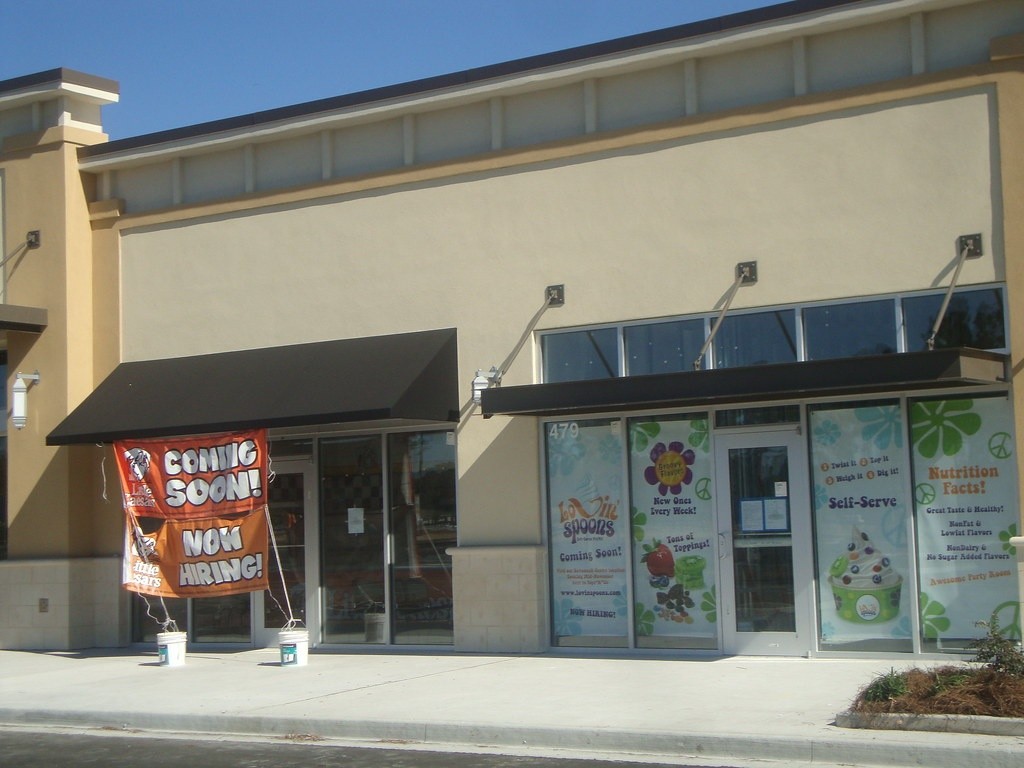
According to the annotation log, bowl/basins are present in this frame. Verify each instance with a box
[831,578,905,623]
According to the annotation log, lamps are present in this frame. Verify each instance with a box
[470,366,501,407]
[12,370,40,430]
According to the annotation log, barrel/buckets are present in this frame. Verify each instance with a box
[363,614,387,643]
[157,620,187,668]
[278,619,309,668]
